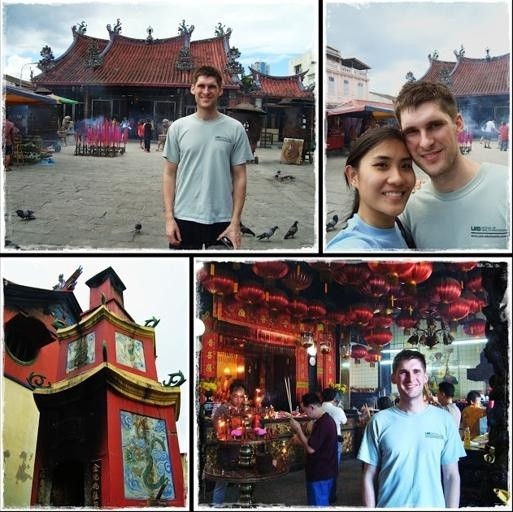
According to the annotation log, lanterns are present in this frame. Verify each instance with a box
[197,262,487,366]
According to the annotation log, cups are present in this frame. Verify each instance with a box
[487,400,494,408]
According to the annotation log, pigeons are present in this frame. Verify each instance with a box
[256,225,279,240]
[274,169,295,184]
[14,208,35,221]
[134,223,142,232]
[48,158,54,164]
[326,214,338,232]
[239,221,255,238]
[284,220,298,239]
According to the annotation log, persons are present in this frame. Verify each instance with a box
[480,116,508,151]
[162,66,254,250]
[5,114,13,171]
[325,128,417,249]
[397,80,509,249]
[356,350,487,509]
[213,382,245,503]
[290,394,337,506]
[322,388,347,464]
[138,118,152,152]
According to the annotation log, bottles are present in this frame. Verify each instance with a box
[464,426,471,447]
